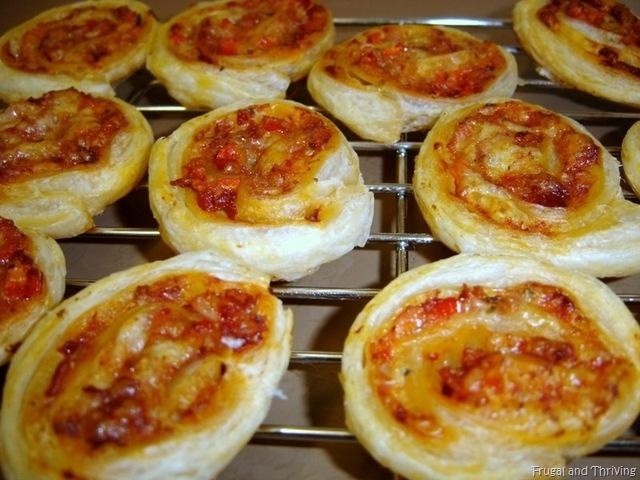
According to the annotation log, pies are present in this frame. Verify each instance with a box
[0,0,640,480]
